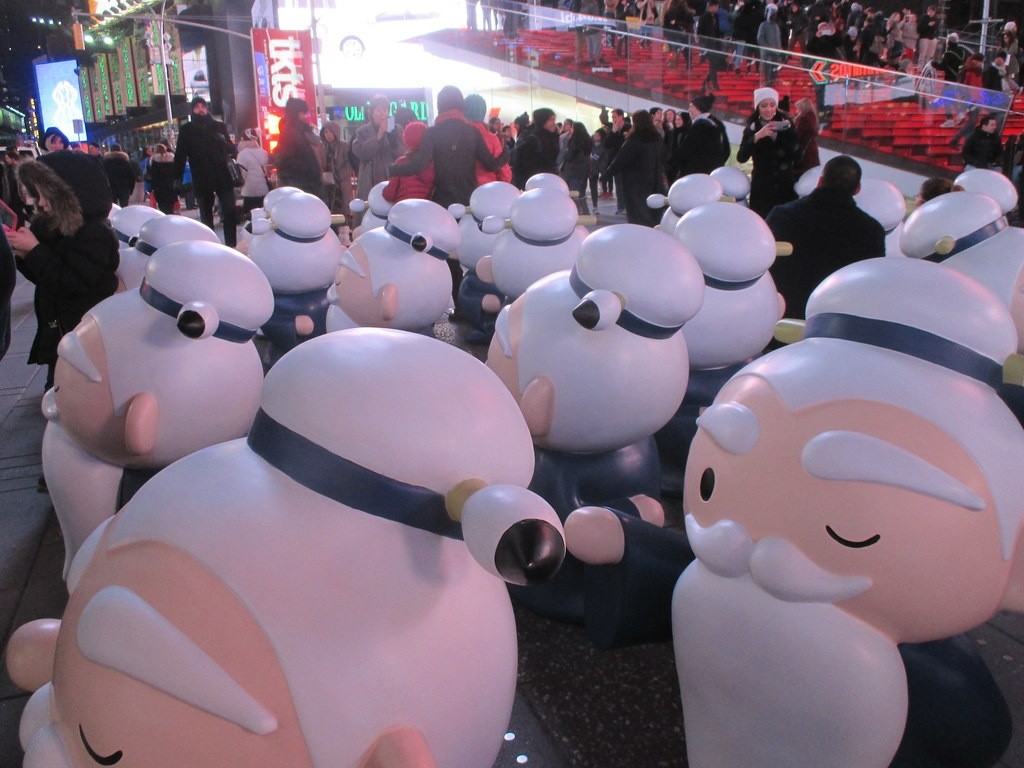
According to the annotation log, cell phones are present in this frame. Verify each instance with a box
[0,199,17,232]
[769,121,789,131]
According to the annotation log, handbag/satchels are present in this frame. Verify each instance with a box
[791,152,805,169]
[226,157,248,188]
[149,192,156,208]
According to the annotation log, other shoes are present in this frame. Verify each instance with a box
[939,120,954,128]
[1017,87,1023,95]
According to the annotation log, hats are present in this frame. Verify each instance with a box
[997,51,1007,61]
[190,97,207,111]
[534,108,555,126]
[753,87,778,110]
[464,96,486,121]
[692,93,715,114]
[948,33,959,44]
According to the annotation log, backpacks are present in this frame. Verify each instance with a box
[869,34,885,55]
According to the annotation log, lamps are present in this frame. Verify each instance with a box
[90,19,98,25]
[85,21,92,27]
[102,10,111,17]
[110,7,119,14]
[118,2,127,11]
[95,14,105,22]
[126,0,135,6]
[133,0,142,4]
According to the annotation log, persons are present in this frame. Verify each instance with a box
[737,87,801,218]
[915,177,965,206]
[765,155,888,351]
[0,327,568,768]
[793,99,819,172]
[353,86,731,342]
[270,99,355,245]
[563,254,1024,768]
[961,116,1024,228]
[39,165,1024,647]
[6,150,120,489]
[466,0,559,40]
[0,97,267,358]
[928,20,1024,149]
[562,0,938,129]
